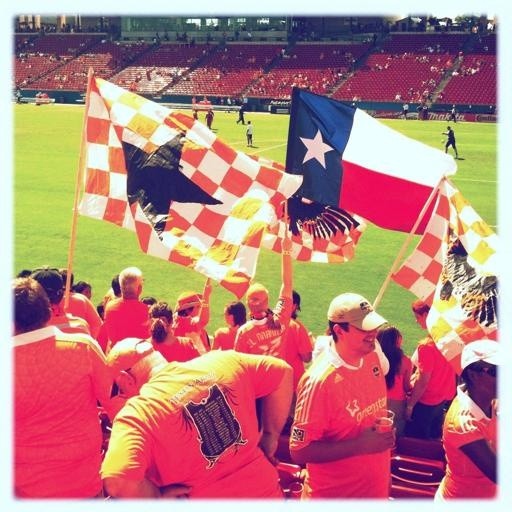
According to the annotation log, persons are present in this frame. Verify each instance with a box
[237,105,246,125]
[423,105,428,119]
[448,105,456,121]
[193,109,198,120]
[14,15,497,107]
[246,121,253,146]
[12,231,498,496]
[205,108,214,129]
[402,102,409,121]
[442,127,458,158]
[416,104,423,120]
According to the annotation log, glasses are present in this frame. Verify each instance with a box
[478,365,497,378]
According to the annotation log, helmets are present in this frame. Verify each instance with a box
[293,291,301,312]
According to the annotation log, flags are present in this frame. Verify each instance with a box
[391,178,499,361]
[75,67,304,298]
[244,153,369,265]
[287,88,457,232]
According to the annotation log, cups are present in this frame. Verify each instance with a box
[288,482,304,500]
[374,409,395,433]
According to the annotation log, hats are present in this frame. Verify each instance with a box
[175,294,202,311]
[459,338,499,376]
[326,293,388,332]
[104,337,157,400]
[30,266,64,298]
